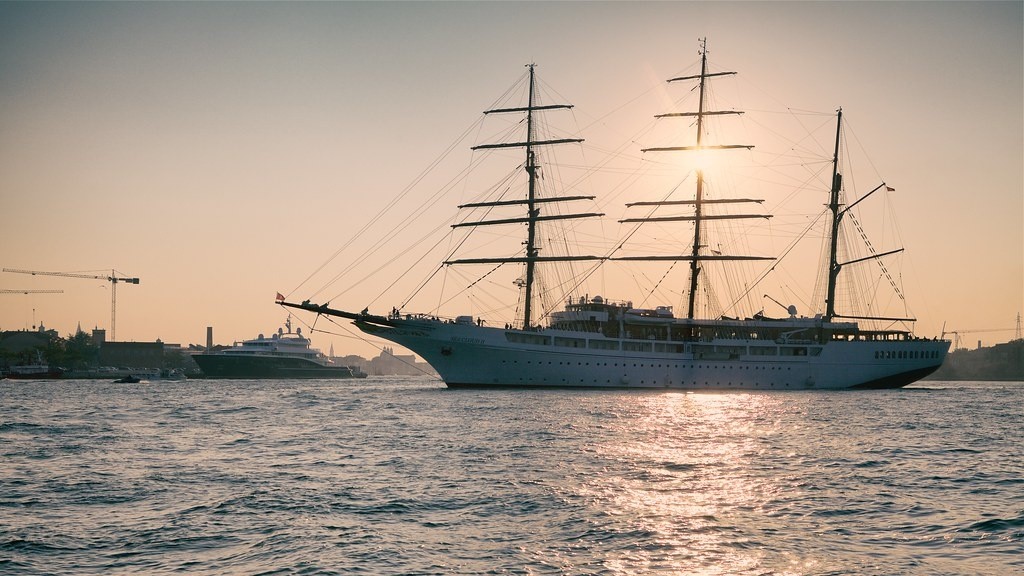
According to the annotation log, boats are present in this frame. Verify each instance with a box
[112,373,141,384]
[1,348,66,380]
[188,315,369,379]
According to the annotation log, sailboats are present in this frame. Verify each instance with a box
[274,34,954,394]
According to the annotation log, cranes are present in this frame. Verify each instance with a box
[0,288,65,295]
[0,267,141,342]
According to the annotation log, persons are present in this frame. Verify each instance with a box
[908,334,939,342]
[393,307,400,319]
[798,349,807,356]
[505,323,512,329]
[431,316,454,324]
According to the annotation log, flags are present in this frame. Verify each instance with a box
[276,293,285,300]
[887,187,895,191]
